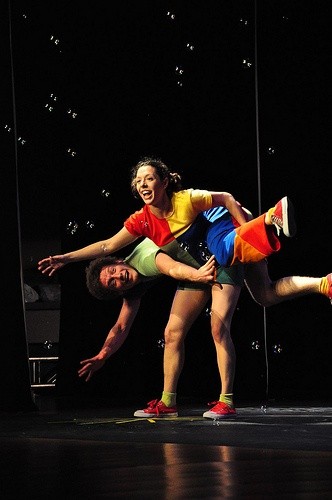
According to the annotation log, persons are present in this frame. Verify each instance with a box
[78,200,332,380]
[37,156,247,419]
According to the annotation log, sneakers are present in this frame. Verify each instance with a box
[269,195,296,238]
[327,273,332,305]
[134,401,178,418]
[203,401,236,419]
